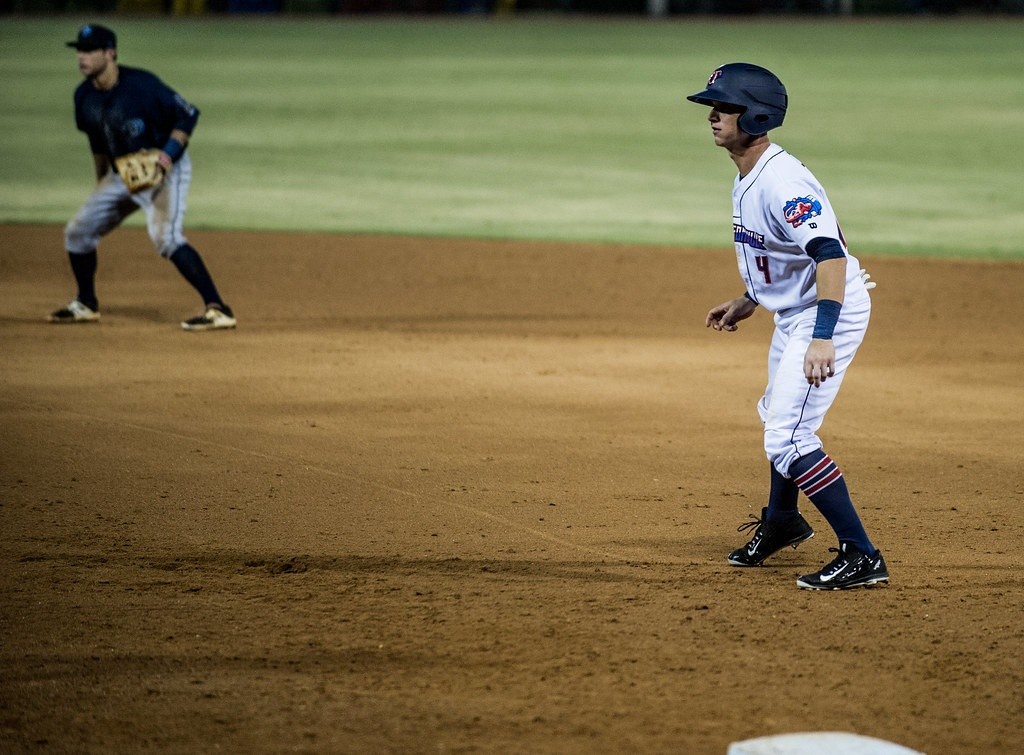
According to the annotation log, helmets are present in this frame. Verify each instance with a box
[686,62,790,137]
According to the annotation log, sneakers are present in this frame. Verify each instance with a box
[46,301,101,322]
[797,544,892,591]
[180,305,237,331]
[728,507,816,566]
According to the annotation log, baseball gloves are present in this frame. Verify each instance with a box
[111,147,172,195]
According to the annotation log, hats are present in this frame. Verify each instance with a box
[65,23,117,52]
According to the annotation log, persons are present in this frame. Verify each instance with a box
[42,24,237,332]
[703,62,892,591]
